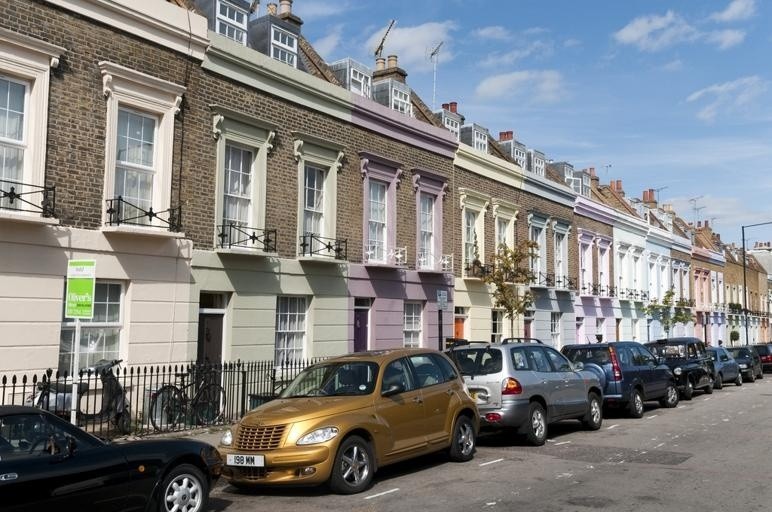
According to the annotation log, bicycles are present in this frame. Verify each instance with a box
[148,363,227,433]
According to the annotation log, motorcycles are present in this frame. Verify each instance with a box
[25,358,132,435]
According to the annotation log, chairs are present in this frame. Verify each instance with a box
[333,369,359,394]
[459,357,502,374]
[365,364,437,395]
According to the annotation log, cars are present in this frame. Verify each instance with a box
[216,348,481,495]
[0,405,224,512]
[705,343,772,389]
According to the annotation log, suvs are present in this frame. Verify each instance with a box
[432,337,717,445]
